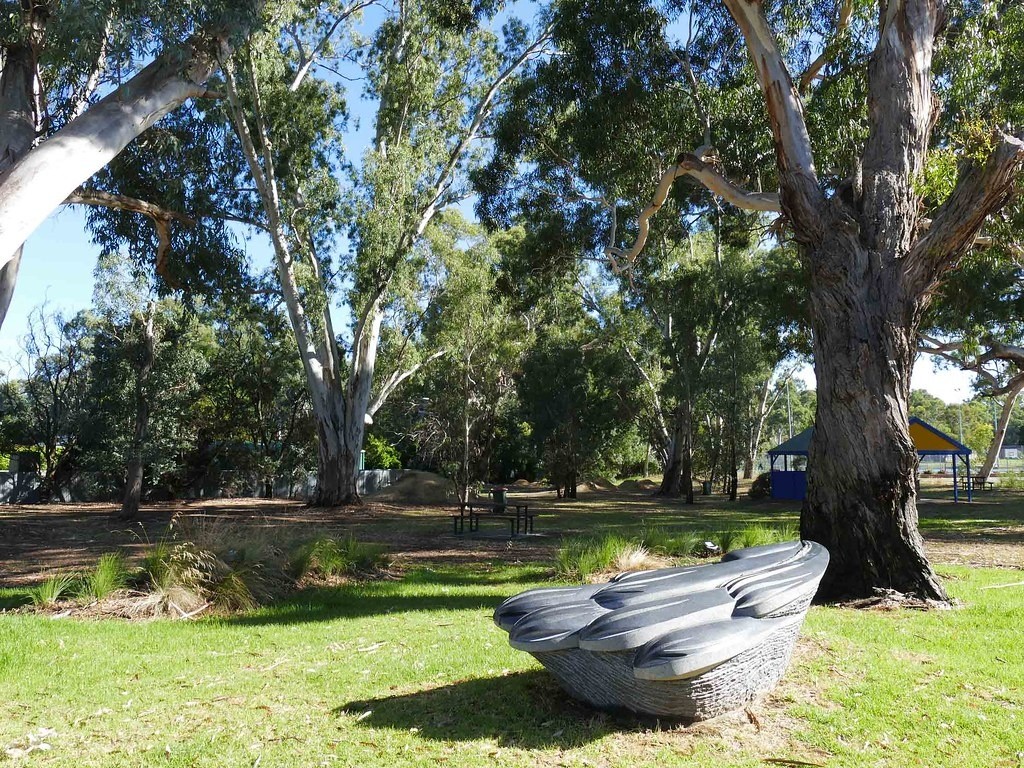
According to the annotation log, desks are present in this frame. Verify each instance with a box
[459,502,532,529]
[961,476,987,491]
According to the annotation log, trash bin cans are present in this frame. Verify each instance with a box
[491,487,509,513]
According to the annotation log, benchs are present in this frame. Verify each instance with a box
[956,481,994,490]
[469,514,539,531]
[448,514,525,536]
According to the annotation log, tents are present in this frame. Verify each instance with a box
[767,416,973,503]
